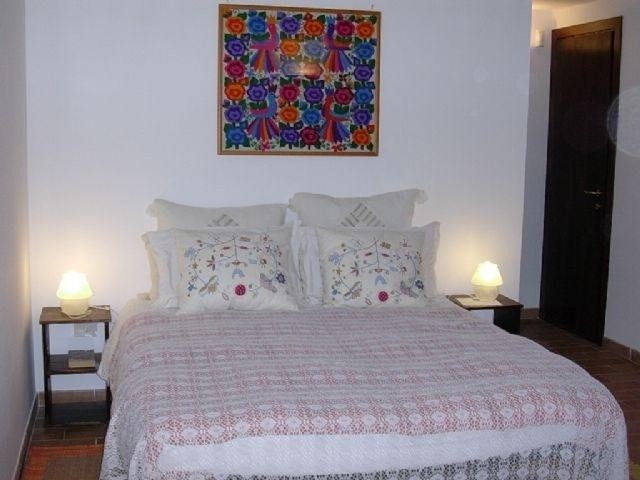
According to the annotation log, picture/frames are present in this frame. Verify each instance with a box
[218,4,381,156]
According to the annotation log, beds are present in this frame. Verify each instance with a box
[105,293,630,479]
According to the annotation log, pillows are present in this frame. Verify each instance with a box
[147,200,291,230]
[290,189,427,309]
[143,221,304,309]
[310,223,441,309]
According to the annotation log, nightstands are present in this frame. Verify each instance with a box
[40,306,111,424]
[446,293,523,335]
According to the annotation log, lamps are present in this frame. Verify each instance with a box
[56,271,94,316]
[471,260,504,301]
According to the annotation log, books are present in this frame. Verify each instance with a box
[69,350,95,368]
